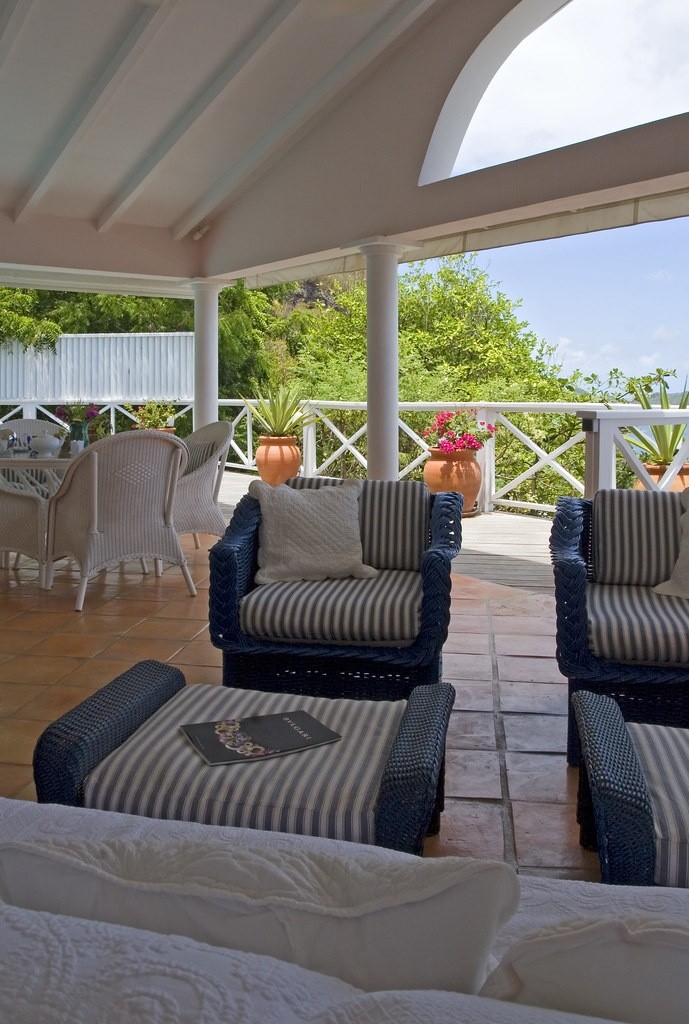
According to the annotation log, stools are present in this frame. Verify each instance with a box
[32,660,456,858]
[569,690,689,888]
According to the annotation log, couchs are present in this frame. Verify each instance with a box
[0,796,689,1024]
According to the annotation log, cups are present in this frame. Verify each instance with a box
[71,440,84,456]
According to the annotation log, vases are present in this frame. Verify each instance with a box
[145,427,177,435]
[422,447,482,513]
[69,422,90,448]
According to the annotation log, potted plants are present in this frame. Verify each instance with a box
[235,379,327,485]
[605,376,689,492]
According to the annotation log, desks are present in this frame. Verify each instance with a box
[0,449,75,496]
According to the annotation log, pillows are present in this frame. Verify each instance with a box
[1,838,522,995]
[247,478,378,584]
[479,915,689,1024]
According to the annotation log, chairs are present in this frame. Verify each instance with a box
[209,477,464,701]
[548,488,689,767]
[0,420,233,610]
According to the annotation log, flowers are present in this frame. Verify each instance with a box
[123,393,180,430]
[55,398,99,437]
[423,405,497,454]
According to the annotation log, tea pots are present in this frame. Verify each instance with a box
[30,429,65,457]
